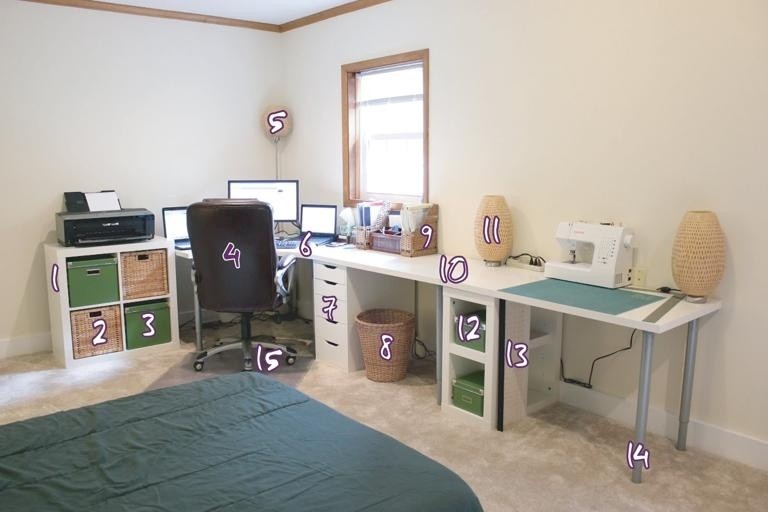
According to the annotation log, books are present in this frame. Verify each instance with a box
[400,203,438,234]
[357,203,373,226]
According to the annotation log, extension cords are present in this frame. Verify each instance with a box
[506,257,545,272]
[253,308,279,316]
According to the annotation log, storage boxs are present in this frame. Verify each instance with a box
[452,367,484,417]
[124,301,170,349]
[67,258,118,308]
[455,310,486,351]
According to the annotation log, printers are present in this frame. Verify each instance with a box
[56,190,154,247]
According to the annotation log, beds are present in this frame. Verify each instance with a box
[0,370,483,511]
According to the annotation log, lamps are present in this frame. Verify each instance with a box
[673,213,724,303]
[474,195,512,265]
[264,106,293,180]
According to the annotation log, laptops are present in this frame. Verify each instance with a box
[289,205,337,247]
[163,207,192,250]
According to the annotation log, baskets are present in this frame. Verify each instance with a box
[69,305,125,361]
[119,249,172,302]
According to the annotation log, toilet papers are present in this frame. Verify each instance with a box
[338,207,356,235]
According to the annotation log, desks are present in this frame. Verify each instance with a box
[179,241,723,482]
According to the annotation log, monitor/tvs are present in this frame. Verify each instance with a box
[228,180,299,222]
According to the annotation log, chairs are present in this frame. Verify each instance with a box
[188,198,296,374]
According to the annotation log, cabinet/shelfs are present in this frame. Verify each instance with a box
[44,236,177,366]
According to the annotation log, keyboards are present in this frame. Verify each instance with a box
[274,240,298,249]
